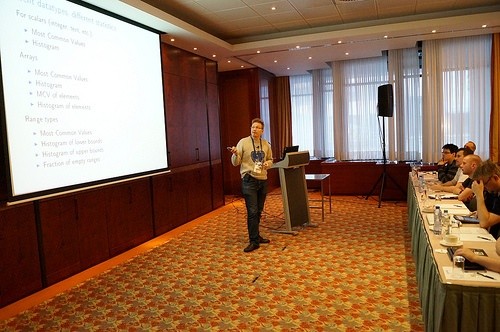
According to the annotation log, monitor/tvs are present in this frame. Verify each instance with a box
[282,145,300,160]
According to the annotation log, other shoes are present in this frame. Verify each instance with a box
[244,243,260,252]
[259,235,270,243]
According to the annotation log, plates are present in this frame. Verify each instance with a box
[439,240,464,247]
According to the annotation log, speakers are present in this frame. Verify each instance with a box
[378,84,394,117]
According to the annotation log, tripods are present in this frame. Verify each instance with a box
[364,116,406,208]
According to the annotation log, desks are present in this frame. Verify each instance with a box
[408,171,500,332]
[304,174,331,222]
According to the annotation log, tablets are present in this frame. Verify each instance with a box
[454,215,479,223]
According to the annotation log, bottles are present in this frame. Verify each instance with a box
[434,205,441,236]
[441,210,451,235]
[412,164,429,207]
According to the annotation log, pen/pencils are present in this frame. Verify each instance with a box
[477,235,493,241]
[477,271,496,280]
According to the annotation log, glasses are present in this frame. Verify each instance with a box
[250,126,263,130]
[441,151,450,154]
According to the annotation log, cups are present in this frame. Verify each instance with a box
[443,235,458,245]
[451,221,460,242]
[453,255,464,276]
[423,172,441,202]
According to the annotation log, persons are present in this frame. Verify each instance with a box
[453,237,500,272]
[453,154,483,212]
[230,118,273,252]
[468,161,500,240]
[464,142,476,153]
[437,144,459,184]
[431,148,474,195]
